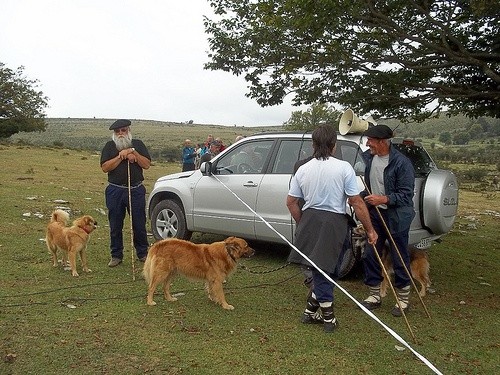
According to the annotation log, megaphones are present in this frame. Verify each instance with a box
[339,109,374,136]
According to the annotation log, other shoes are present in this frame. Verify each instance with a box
[108,257,123,267]
[361,295,381,310]
[391,302,411,317]
[139,256,146,262]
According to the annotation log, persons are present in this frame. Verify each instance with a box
[182,134,245,172]
[347,123,416,316]
[286,125,379,330]
[100,119,151,267]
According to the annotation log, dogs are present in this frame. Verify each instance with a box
[142,237,256,310]
[351,224,436,297]
[46,209,98,277]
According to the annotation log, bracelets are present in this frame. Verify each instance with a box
[131,148,135,153]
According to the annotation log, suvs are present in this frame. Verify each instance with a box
[146,130,460,280]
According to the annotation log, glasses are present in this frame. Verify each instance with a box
[114,129,126,133]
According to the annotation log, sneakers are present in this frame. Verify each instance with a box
[323,317,338,332]
[302,311,324,324]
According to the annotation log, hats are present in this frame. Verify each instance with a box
[109,119,131,130]
[362,125,393,139]
